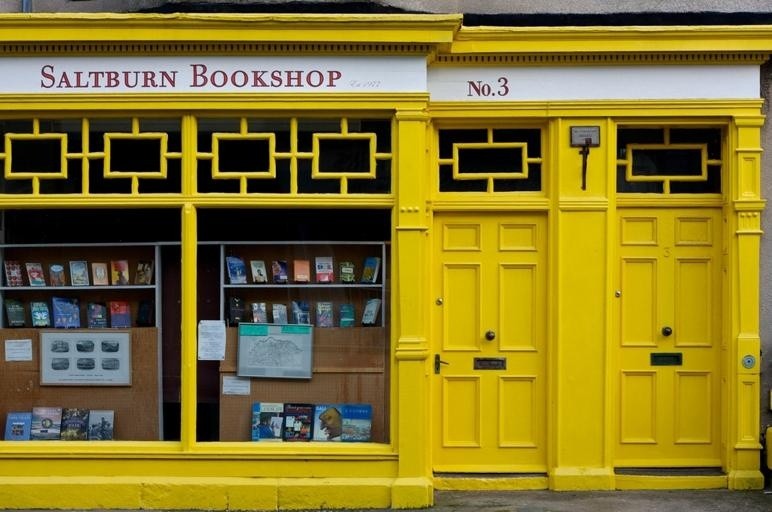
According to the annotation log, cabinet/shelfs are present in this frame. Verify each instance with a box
[0,242,162,329]
[219,240,385,327]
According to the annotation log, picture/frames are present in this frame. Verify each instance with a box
[238,322,313,379]
[39,331,133,388]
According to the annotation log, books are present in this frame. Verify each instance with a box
[282,402,315,443]
[89,409,115,441]
[249,400,285,441]
[3,412,32,441]
[226,254,382,328]
[342,401,372,443]
[59,408,89,441]
[4,259,157,329]
[30,406,62,440]
[312,403,343,443]
